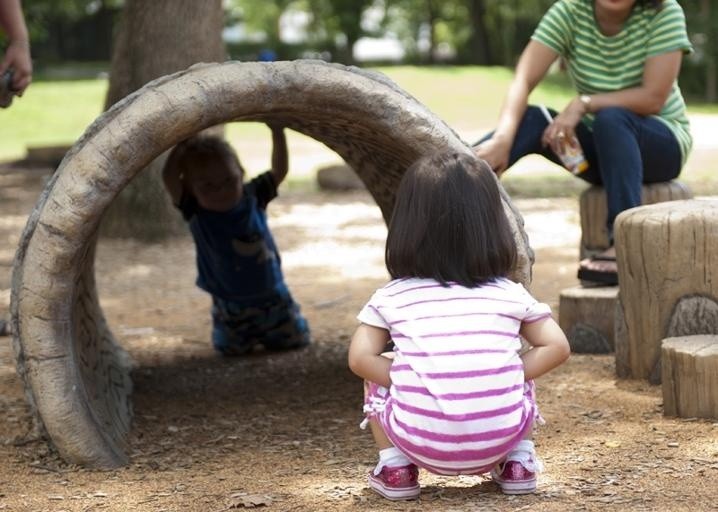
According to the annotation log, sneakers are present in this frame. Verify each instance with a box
[367,462,421,501]
[491,458,538,495]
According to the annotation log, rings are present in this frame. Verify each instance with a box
[556,132,567,138]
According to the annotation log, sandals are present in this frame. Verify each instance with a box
[577,253,620,285]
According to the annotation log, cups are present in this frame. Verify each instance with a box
[551,129,590,176]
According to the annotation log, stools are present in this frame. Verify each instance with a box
[613,197,718,386]
[576,178,688,286]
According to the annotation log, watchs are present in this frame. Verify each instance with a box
[578,93,593,111]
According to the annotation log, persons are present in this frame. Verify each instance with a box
[0,0,34,98]
[471,0,693,282]
[162,119,309,357]
[348,150,572,500]
